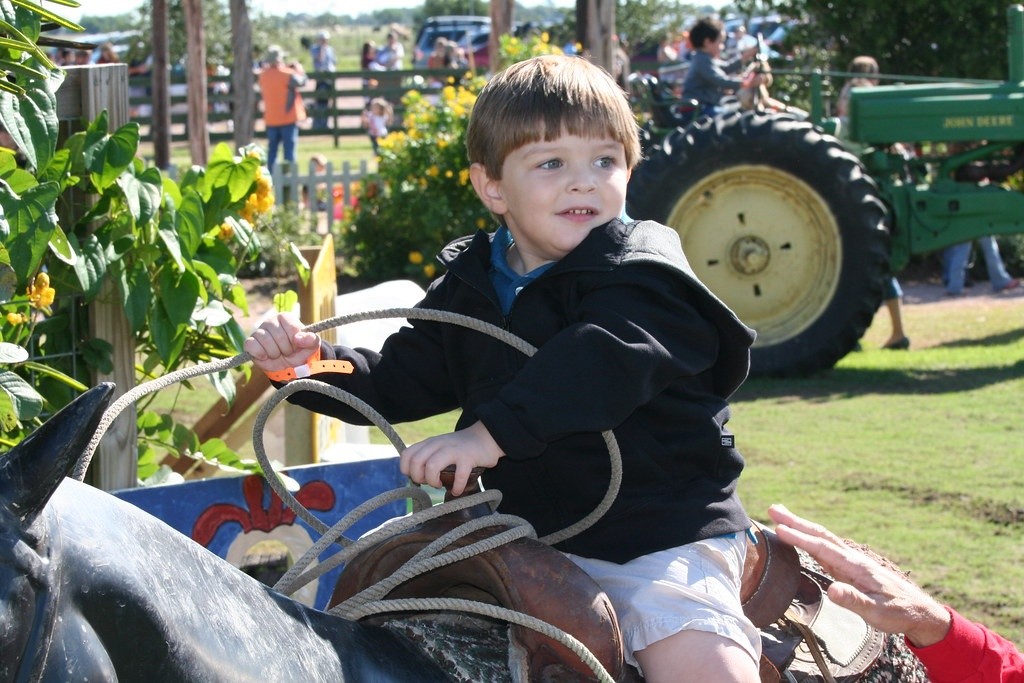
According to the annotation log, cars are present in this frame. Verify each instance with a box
[412,17,491,69]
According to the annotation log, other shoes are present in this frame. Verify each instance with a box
[1004,279,1020,290]
[882,336,909,350]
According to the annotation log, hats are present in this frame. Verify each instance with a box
[262,45,291,63]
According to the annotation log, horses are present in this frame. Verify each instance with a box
[0,381,931,682]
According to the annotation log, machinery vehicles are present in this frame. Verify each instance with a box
[626,0,1024,379]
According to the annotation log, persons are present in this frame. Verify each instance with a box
[258,46,308,169]
[45,43,119,66]
[770,504,1024,682]
[940,141,1019,293]
[128,54,152,74]
[846,56,880,85]
[834,79,909,350]
[363,34,404,105]
[236,56,766,681]
[657,31,678,63]
[429,37,466,68]
[311,31,336,128]
[682,21,769,119]
[363,98,393,149]
[735,35,774,107]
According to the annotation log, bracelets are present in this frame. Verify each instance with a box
[265,344,355,382]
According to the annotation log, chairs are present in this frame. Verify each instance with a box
[627,72,699,128]
